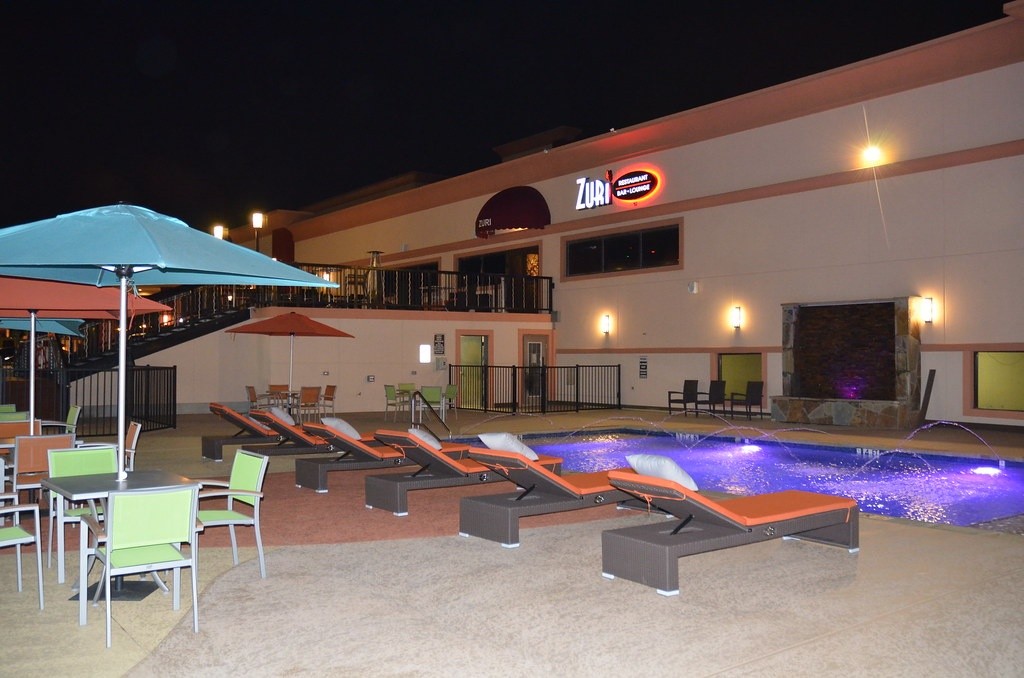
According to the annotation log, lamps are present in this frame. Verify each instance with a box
[604,315,609,333]
[925,298,932,323]
[734,307,740,328]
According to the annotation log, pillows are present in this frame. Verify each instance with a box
[271,407,295,426]
[408,428,442,451]
[625,454,699,491]
[247,416,271,430]
[321,418,361,440]
[478,433,539,461]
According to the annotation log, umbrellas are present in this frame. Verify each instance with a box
[0,201,338,481]
[224,312,355,411]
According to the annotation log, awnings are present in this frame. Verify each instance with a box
[474,186,551,239]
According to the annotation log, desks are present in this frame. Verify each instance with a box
[40,469,203,607]
[0,440,83,520]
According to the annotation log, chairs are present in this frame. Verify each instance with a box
[668,380,764,422]
[200,403,563,517]
[0,404,269,647]
[345,270,370,298]
[385,383,458,424]
[459,447,674,548]
[241,410,386,456]
[601,471,859,597]
[244,380,337,425]
